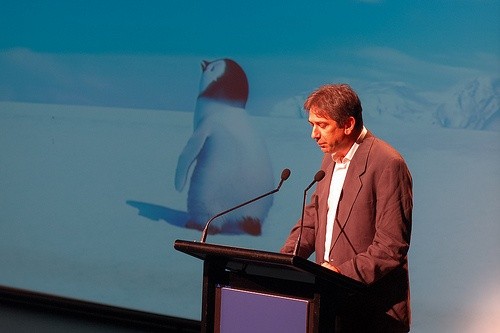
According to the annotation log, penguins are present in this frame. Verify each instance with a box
[175,58,274,236]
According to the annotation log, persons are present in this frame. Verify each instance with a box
[280,84,414,333]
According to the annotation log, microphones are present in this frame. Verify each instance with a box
[201,168,291,243]
[292,170,326,256]
